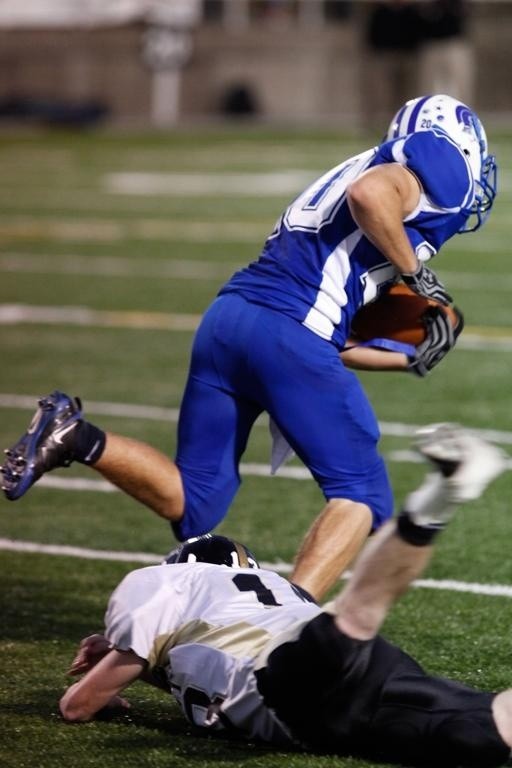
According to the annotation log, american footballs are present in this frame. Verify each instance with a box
[348,277,457,349]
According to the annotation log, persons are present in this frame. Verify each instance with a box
[3,86,498,604]
[60,419,512,765]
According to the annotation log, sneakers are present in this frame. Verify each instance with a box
[2,392,84,501]
[412,421,511,501]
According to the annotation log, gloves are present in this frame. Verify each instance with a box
[401,260,455,309]
[406,303,458,380]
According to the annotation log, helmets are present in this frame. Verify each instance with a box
[383,93,498,234]
[161,533,259,569]
